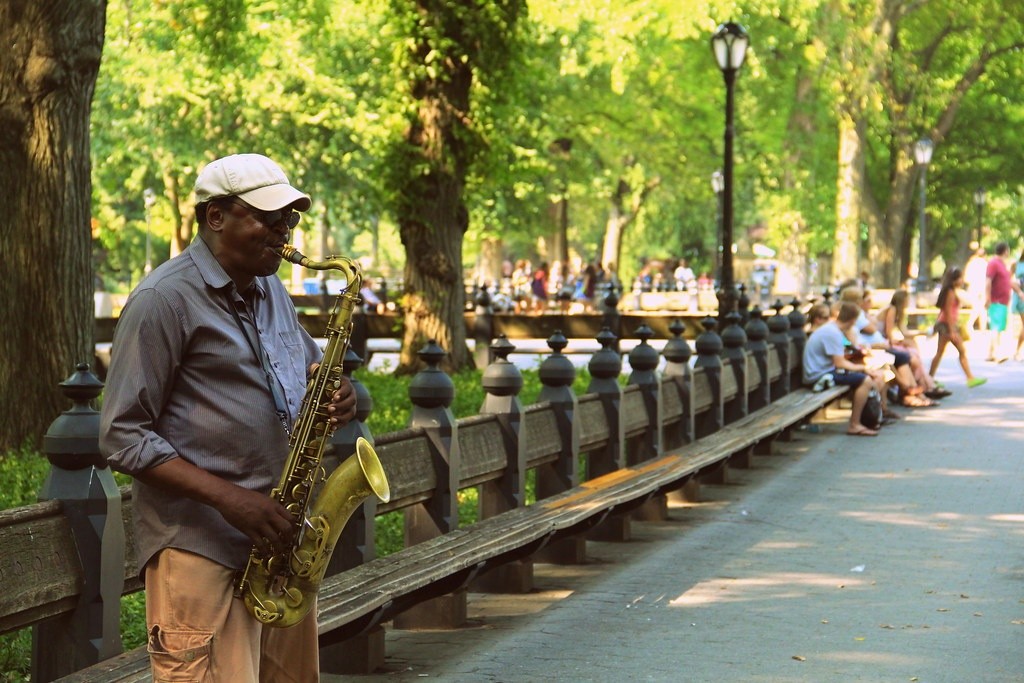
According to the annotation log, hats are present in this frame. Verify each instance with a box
[191,153,313,216]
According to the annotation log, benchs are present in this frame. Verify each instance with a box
[52,385,850,683]
[367,346,697,361]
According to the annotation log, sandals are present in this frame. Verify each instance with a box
[926,387,954,398]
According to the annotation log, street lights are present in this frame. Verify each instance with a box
[710,166,725,291]
[143,187,155,277]
[914,131,934,308]
[709,14,752,346]
[973,186,987,248]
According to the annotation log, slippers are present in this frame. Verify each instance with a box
[848,427,879,437]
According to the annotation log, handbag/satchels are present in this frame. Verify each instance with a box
[862,389,885,431]
[956,312,973,343]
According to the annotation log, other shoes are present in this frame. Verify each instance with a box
[968,375,987,389]
[985,357,995,363]
[935,381,946,387]
[881,408,900,421]
[998,357,1009,365]
[902,393,943,408]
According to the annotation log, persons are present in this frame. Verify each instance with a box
[499,255,620,299]
[804,278,954,425]
[359,281,382,312]
[1008,248,1024,362]
[965,249,988,311]
[672,257,696,291]
[801,302,879,433]
[96,152,356,681]
[926,265,987,389]
[698,269,714,288]
[984,243,1024,363]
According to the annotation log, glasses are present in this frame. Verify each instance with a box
[226,198,303,233]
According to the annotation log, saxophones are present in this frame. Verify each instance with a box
[229,244,396,631]
